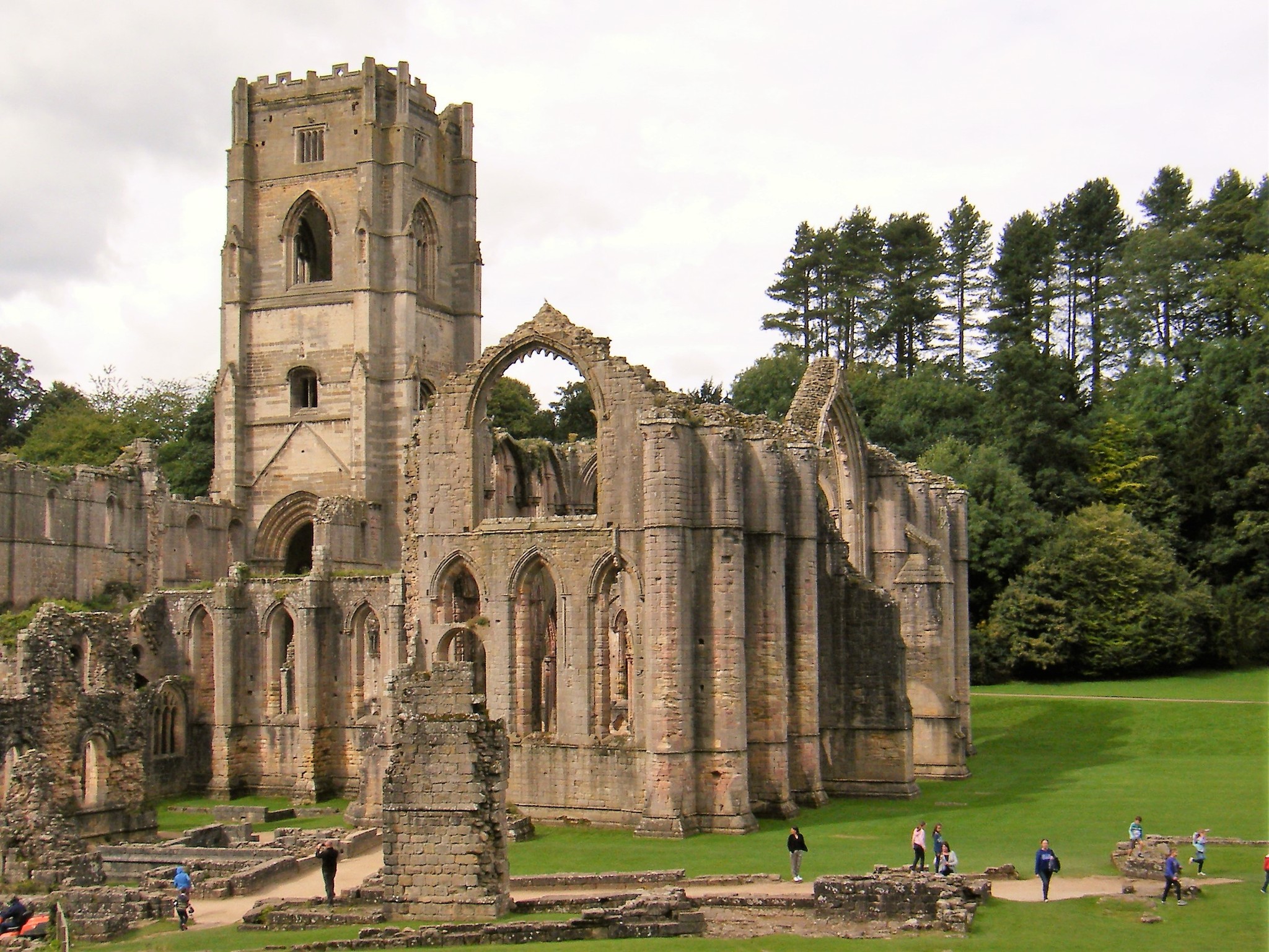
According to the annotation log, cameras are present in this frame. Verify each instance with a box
[322,844,325,846]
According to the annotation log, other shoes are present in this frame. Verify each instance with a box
[1188,856,1193,864]
[181,924,188,930]
[1197,872,1206,876]
[1177,901,1187,905]
[797,875,802,880]
[1162,901,1167,904]
[1138,854,1144,858]
[1260,889,1266,893]
[1045,899,1048,902]
[794,877,799,882]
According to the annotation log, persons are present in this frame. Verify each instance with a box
[1260,854,1269,893]
[0,896,27,935]
[940,842,958,876]
[1034,839,1056,902]
[316,841,339,907]
[173,886,192,930]
[911,821,927,873]
[932,823,943,873]
[175,867,194,904]
[787,826,808,881]
[1161,848,1188,905]
[1127,816,1144,857]
[1188,829,1206,876]
